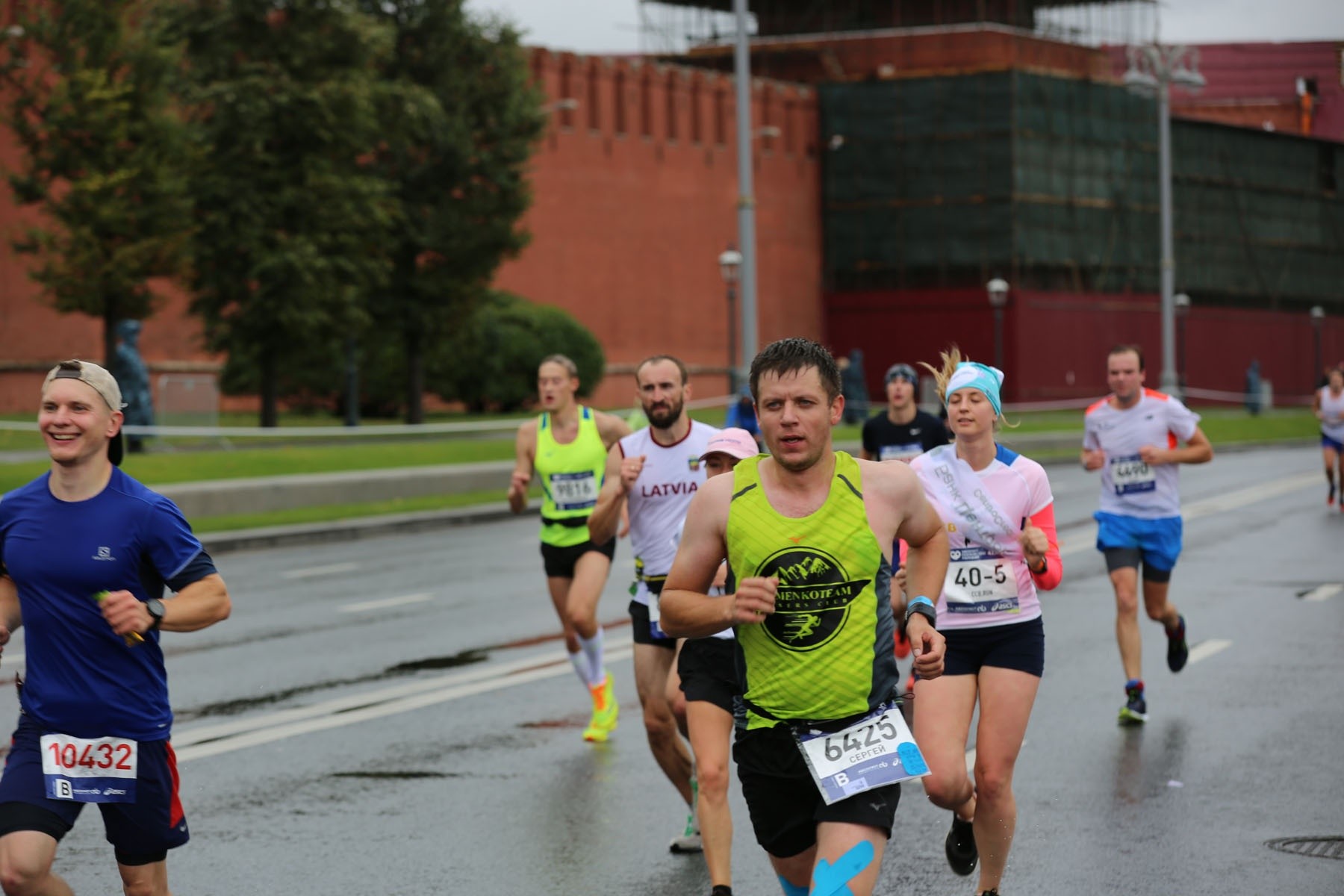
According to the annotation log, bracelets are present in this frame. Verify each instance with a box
[905,595,938,613]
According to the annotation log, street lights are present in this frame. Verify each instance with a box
[717,243,743,396]
[1174,291,1191,388]
[1120,29,1204,405]
[985,278,1008,374]
[1310,305,1324,389]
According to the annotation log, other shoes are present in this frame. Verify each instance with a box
[945,786,978,877]
[982,888,999,896]
[1117,687,1148,723]
[1328,486,1335,504]
[1165,616,1188,674]
[1340,497,1344,512]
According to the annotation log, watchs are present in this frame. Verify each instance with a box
[147,598,165,629]
[1026,555,1047,575]
[904,604,939,629]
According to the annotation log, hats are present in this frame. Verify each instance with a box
[42,358,128,466]
[946,361,1004,416]
[884,364,918,393]
[697,427,759,462]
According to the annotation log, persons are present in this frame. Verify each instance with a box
[1078,343,1215,723]
[659,337,953,896]
[725,384,768,453]
[0,359,233,896]
[587,355,737,855]
[860,362,948,700]
[1312,366,1344,516]
[507,353,639,745]
[676,426,766,896]
[111,319,158,453]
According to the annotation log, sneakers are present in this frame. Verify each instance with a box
[689,774,702,835]
[669,813,703,853]
[582,716,617,742]
[588,670,618,727]
